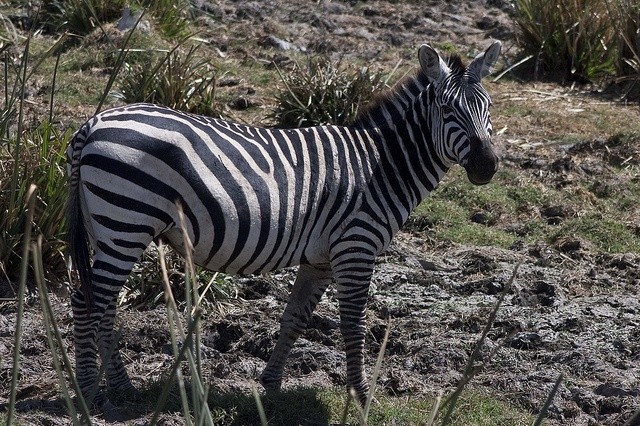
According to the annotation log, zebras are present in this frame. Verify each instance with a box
[62,38,503,417]
